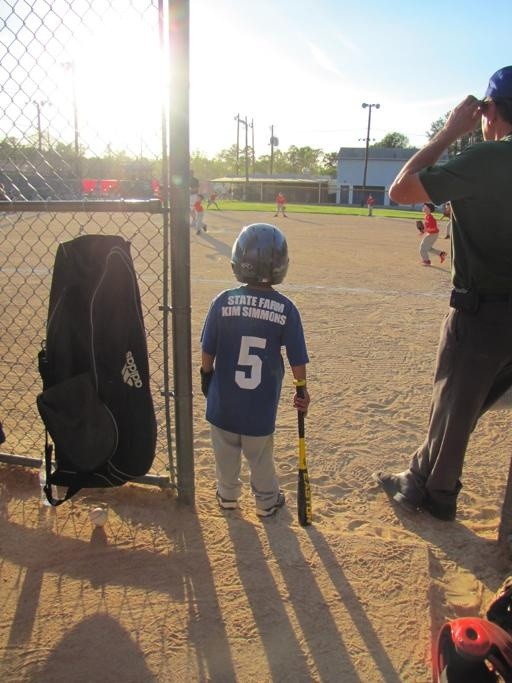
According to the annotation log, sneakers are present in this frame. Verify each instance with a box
[440,251,448,263]
[197,230,202,234]
[215,488,242,510]
[204,225,208,232]
[421,260,431,266]
[256,489,285,516]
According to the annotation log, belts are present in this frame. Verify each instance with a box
[477,291,511,303]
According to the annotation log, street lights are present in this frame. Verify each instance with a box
[31,99,45,150]
[360,102,380,207]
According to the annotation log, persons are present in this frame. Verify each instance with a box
[274,191,286,216]
[418,203,447,263]
[369,63,511,517]
[442,204,454,238]
[367,195,376,216]
[200,222,310,515]
[208,189,222,210]
[196,194,207,233]
[190,169,199,228]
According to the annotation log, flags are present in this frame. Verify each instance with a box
[153,176,163,205]
[100,179,115,191]
[82,177,96,191]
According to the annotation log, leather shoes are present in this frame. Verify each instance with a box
[371,468,421,512]
[424,494,458,521]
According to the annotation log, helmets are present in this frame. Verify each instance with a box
[230,223,289,285]
[432,618,511,681]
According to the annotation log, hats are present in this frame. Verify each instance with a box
[424,203,436,213]
[481,65,510,105]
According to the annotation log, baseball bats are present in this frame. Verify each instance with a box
[292,379,313,525]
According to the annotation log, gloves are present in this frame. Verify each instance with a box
[199,366,212,397]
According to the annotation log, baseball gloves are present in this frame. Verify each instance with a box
[415,220,424,232]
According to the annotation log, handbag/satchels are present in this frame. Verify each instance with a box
[38,234,159,506]
